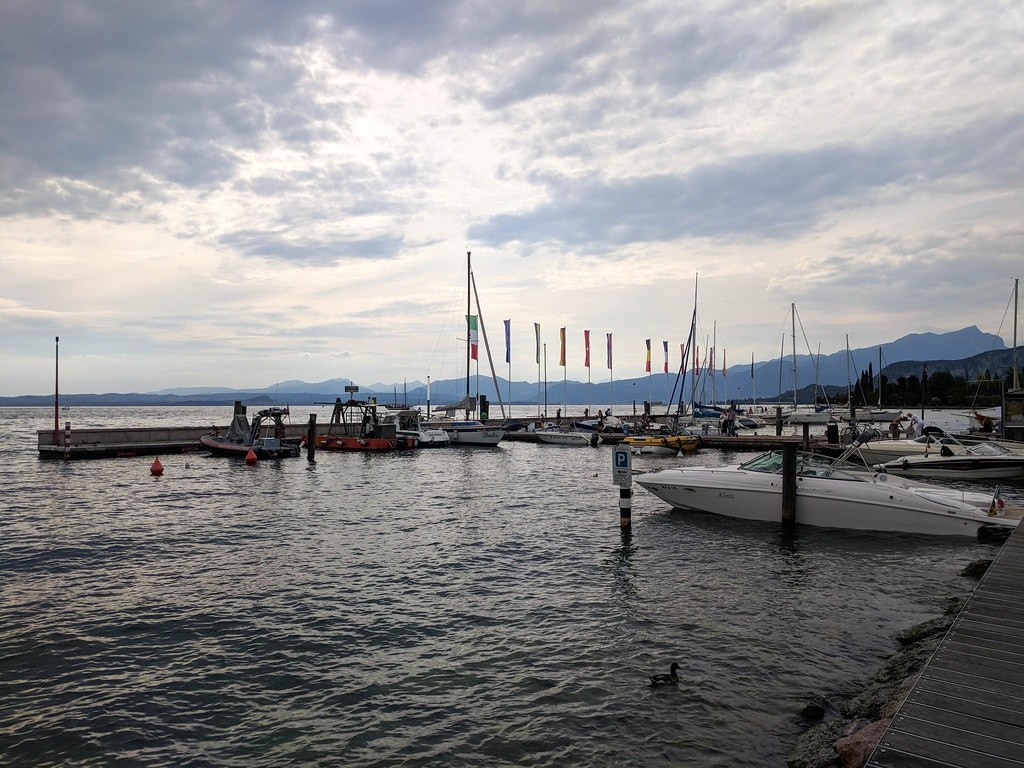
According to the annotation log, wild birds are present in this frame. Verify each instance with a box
[649,663,682,685]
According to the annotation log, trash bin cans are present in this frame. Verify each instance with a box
[827,425,841,444]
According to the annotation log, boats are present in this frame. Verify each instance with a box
[301,396,450,453]
[846,426,1024,479]
[200,401,301,459]
[633,428,1024,540]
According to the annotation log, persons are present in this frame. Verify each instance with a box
[598,410,603,423]
[727,407,736,436]
[892,416,908,439]
[605,408,612,416]
[481,410,488,425]
[908,413,924,438]
[720,410,729,436]
[598,422,609,433]
[643,409,650,432]
[556,410,560,418]
[749,407,752,413]
[584,408,588,419]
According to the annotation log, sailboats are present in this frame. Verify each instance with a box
[420,252,508,448]
[520,271,904,445]
[951,278,1021,430]
[386,377,411,410]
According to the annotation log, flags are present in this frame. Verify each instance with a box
[722,351,726,376]
[696,347,699,375]
[680,344,684,374]
[534,323,540,363]
[606,334,612,368]
[750,354,754,378]
[465,315,478,360]
[646,340,650,372]
[584,331,590,367]
[664,342,668,373]
[709,349,712,374]
[504,320,510,362]
[560,328,565,367]
